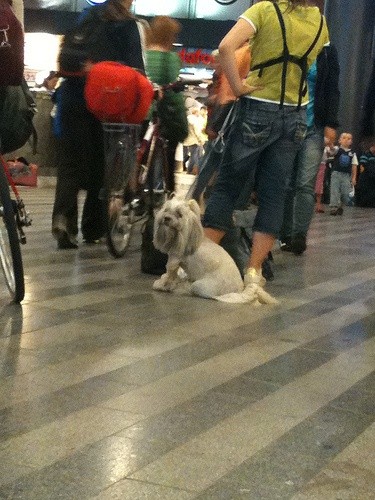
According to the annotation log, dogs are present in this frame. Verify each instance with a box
[152,192,280,305]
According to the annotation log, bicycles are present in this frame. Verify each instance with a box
[80,66,205,260]
[0,149,34,305]
